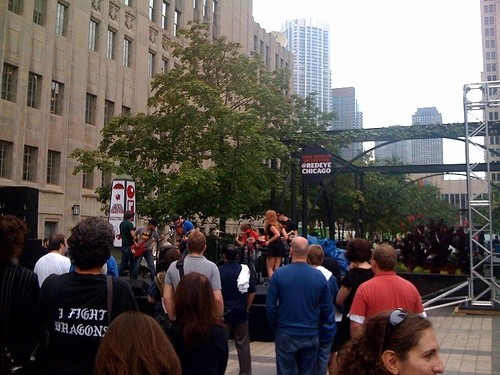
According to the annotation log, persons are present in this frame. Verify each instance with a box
[347,242,427,342]
[159,272,229,375]
[33,210,500,286]
[162,230,224,323]
[307,244,345,375]
[0,214,41,375]
[217,244,256,375]
[41,217,141,375]
[328,308,445,375]
[266,235,334,375]
[336,238,376,352]
[94,311,182,375]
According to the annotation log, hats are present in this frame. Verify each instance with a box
[149,219,158,227]
[172,214,179,222]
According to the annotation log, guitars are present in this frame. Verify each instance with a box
[176,230,192,251]
[129,235,161,257]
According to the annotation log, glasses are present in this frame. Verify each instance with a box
[383,307,408,351]
